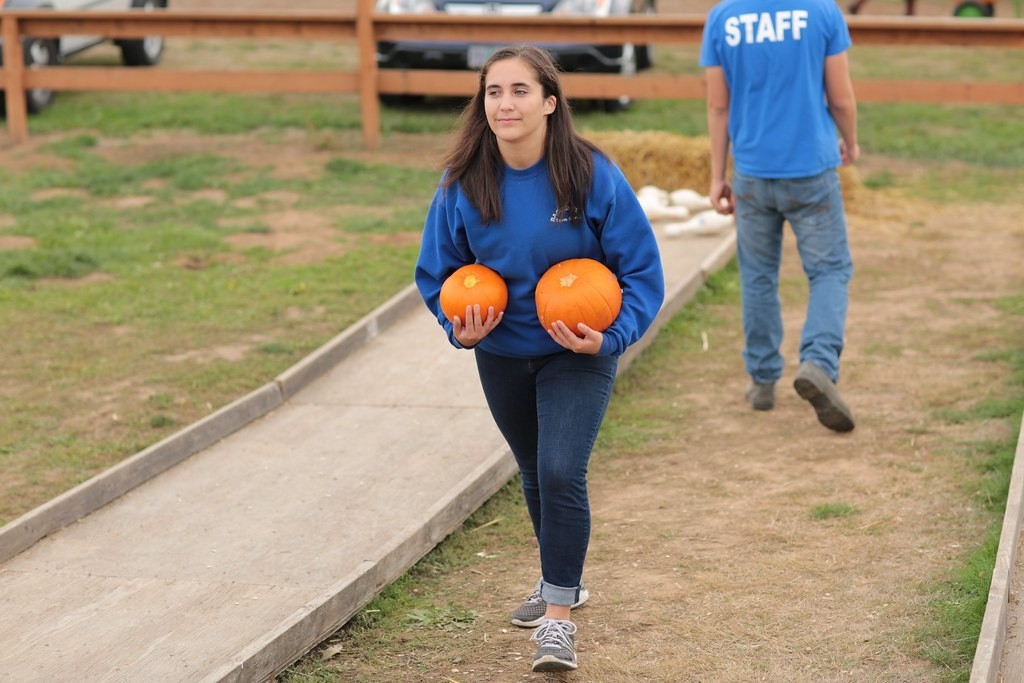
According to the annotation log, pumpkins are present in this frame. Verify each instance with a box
[534,257,622,338]
[439,264,508,327]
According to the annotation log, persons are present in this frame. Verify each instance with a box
[415,45,665,670]
[698,0,857,433]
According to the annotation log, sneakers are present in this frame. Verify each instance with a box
[528,619,578,672]
[511,577,589,626]
[746,381,776,409]
[793,361,855,431]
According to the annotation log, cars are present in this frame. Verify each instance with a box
[373,0,641,113]
[0,0,169,116]
